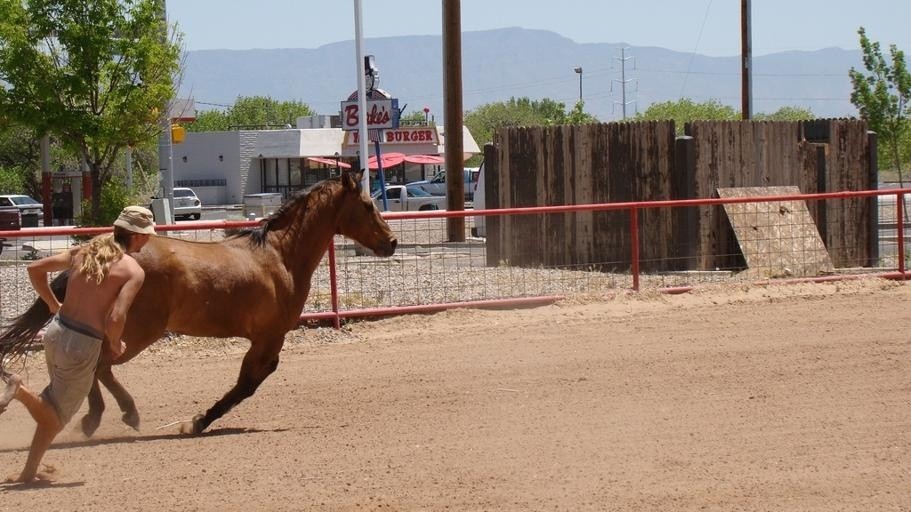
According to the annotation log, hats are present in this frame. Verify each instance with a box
[114,206,157,236]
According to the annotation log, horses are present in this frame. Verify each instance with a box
[0,166,399,438]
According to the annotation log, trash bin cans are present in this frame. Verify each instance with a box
[152,198,173,235]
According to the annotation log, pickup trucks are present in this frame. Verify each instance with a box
[1,206,22,255]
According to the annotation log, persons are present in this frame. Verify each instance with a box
[0,204,159,481]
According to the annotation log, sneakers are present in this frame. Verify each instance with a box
[0,373,22,413]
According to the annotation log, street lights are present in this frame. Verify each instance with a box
[574,66,583,99]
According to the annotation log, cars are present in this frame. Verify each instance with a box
[150,187,202,222]
[369,159,488,238]
[1,194,44,220]
